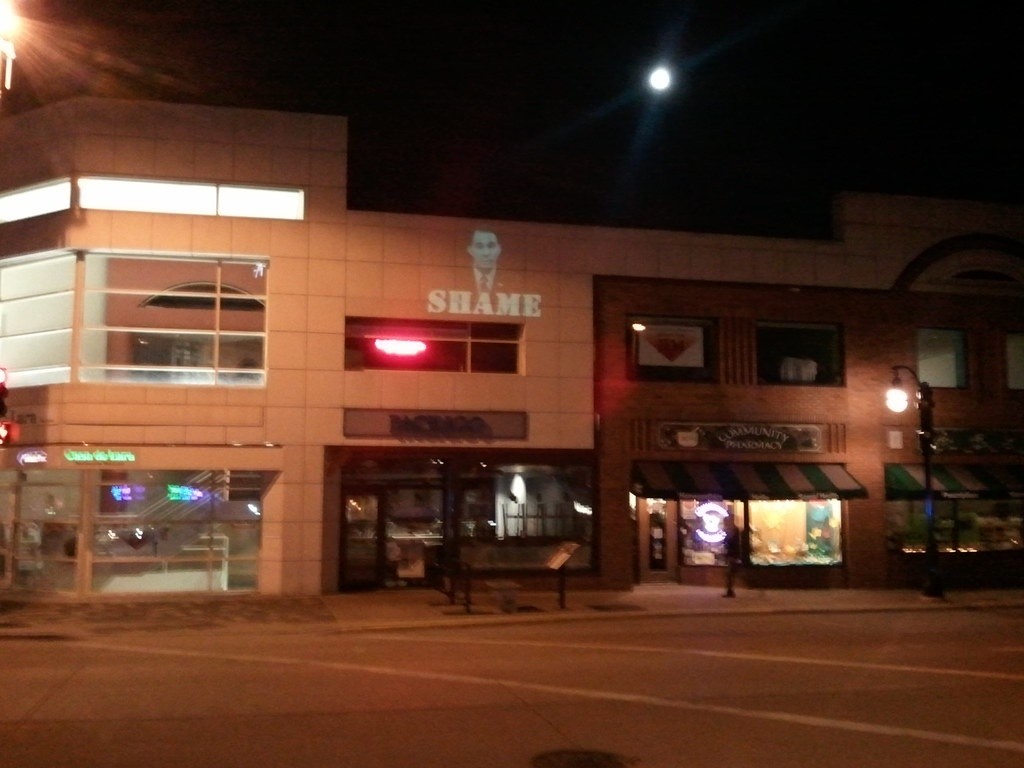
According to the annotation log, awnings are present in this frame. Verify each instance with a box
[627,458,867,502]
[883,461,1024,499]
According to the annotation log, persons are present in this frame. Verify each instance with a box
[720,525,738,598]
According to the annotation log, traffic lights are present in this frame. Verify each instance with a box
[0,370,9,417]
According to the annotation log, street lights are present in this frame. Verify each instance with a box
[886,364,945,597]
